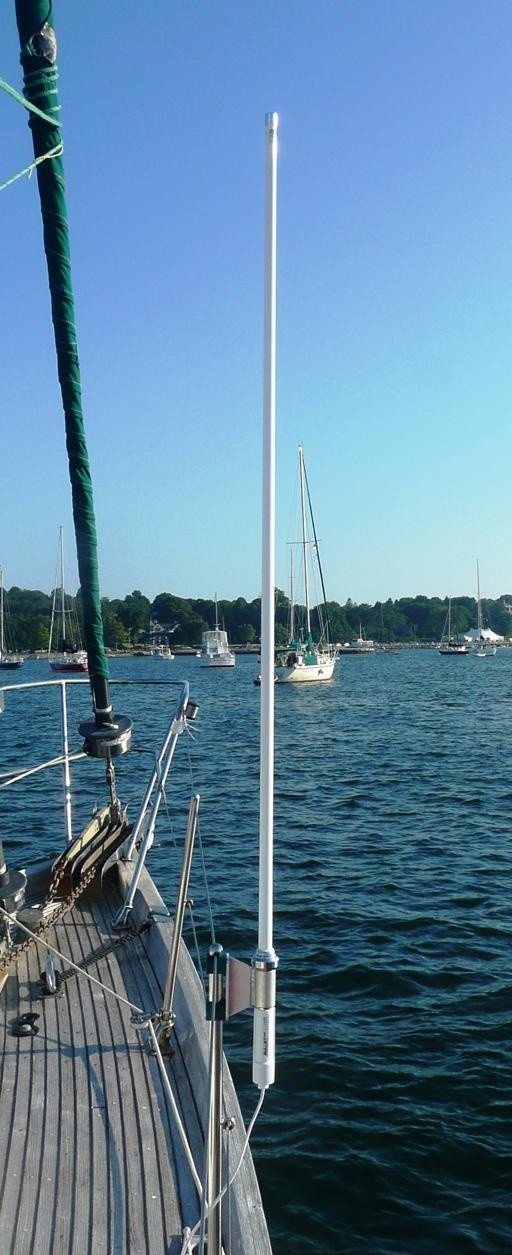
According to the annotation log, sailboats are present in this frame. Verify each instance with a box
[350,621,374,647]
[46,526,89,672]
[436,559,497,657]
[1,571,24,670]
[194,594,234,667]
[255,444,336,683]
[2,3,287,1250]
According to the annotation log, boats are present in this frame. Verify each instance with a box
[152,645,175,660]
